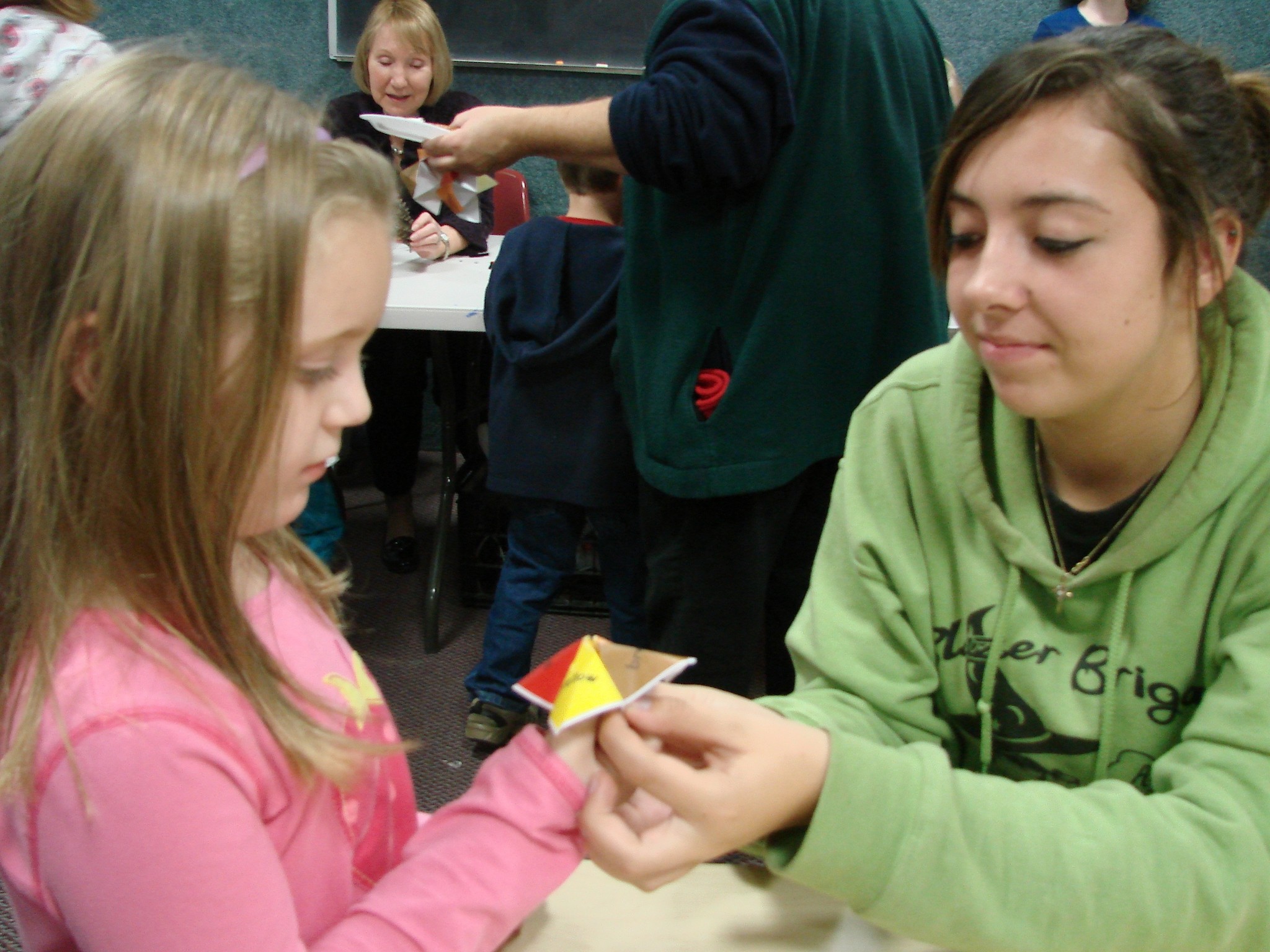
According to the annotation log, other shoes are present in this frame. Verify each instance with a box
[386,514,418,575]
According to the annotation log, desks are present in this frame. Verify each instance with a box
[375,230,509,654]
[501,860,942,952]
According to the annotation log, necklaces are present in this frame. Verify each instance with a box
[1031,418,1191,613]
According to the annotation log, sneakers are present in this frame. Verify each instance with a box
[464,697,525,749]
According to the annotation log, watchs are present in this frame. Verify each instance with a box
[437,230,450,261]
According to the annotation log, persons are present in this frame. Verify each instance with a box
[579,22,1270,952]
[423,1,958,696]
[1029,0,1173,43]
[0,38,664,952]
[464,158,652,742]
[0,0,115,134]
[317,1,495,572]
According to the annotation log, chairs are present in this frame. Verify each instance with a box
[493,166,529,236]
